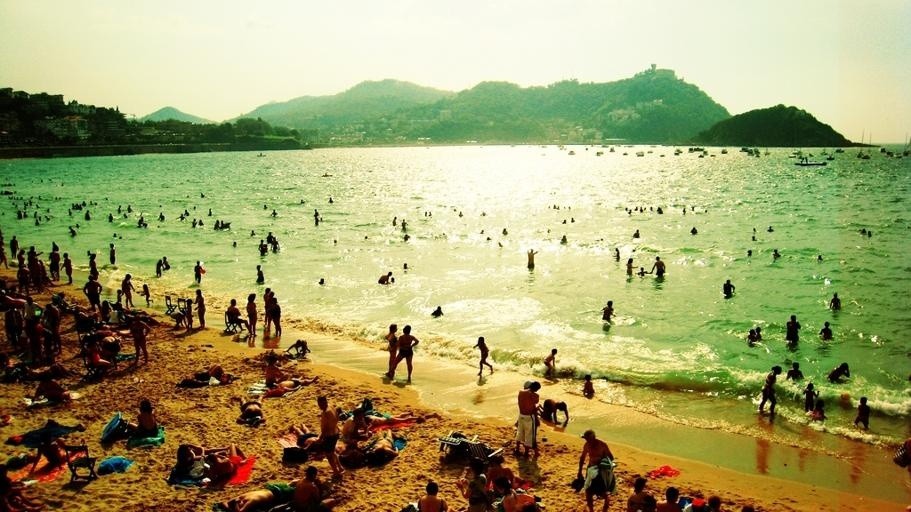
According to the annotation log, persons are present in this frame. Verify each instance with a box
[295,193,413,288]
[5,351,399,512]
[425,202,872,322]
[0,194,286,407]
[747,290,872,432]
[384,307,557,510]
[575,374,756,511]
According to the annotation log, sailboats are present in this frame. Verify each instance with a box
[786,126,910,161]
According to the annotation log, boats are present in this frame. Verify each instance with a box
[564,144,775,159]
[795,158,829,167]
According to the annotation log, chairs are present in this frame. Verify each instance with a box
[177,298,194,319]
[81,351,100,380]
[224,311,238,333]
[460,438,504,471]
[63,445,99,484]
[164,295,177,314]
[438,430,480,459]
